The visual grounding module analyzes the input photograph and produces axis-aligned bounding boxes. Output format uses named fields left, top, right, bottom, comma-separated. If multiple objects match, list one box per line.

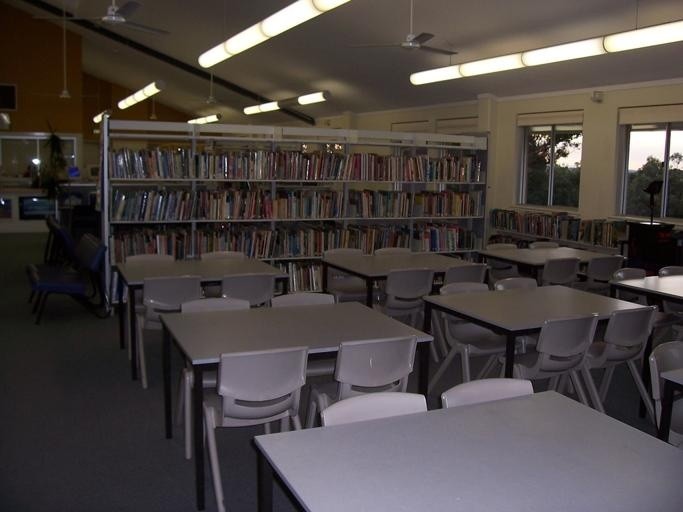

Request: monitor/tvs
left=0, top=84, right=17, bottom=112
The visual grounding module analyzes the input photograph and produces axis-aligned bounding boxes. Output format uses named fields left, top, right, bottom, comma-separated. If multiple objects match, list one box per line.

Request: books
left=109, top=141, right=489, bottom=291
left=489, top=207, right=627, bottom=249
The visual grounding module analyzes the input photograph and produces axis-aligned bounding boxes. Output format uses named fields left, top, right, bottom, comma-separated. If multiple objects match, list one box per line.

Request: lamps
left=116, top=78, right=167, bottom=111
left=59, top=13, right=73, bottom=99
left=243, top=99, right=280, bottom=116
left=297, top=89, right=330, bottom=106
left=185, top=113, right=223, bottom=124
left=196, top=1, right=351, bottom=70
left=408, top=0, right=682, bottom=88
left=93, top=108, right=113, bottom=124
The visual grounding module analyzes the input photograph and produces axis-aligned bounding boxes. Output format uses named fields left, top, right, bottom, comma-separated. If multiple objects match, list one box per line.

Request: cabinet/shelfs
left=487, top=207, right=628, bottom=276
left=104, top=113, right=489, bottom=305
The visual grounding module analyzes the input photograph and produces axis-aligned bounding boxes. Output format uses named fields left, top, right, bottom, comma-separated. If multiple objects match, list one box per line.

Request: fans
left=32, top=0, right=170, bottom=36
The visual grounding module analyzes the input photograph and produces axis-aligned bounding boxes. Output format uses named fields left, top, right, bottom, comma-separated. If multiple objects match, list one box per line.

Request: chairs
left=124, top=242, right=683, bottom=512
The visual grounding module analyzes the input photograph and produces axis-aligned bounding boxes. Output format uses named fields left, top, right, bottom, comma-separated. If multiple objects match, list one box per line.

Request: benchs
left=26, top=212, right=105, bottom=325
